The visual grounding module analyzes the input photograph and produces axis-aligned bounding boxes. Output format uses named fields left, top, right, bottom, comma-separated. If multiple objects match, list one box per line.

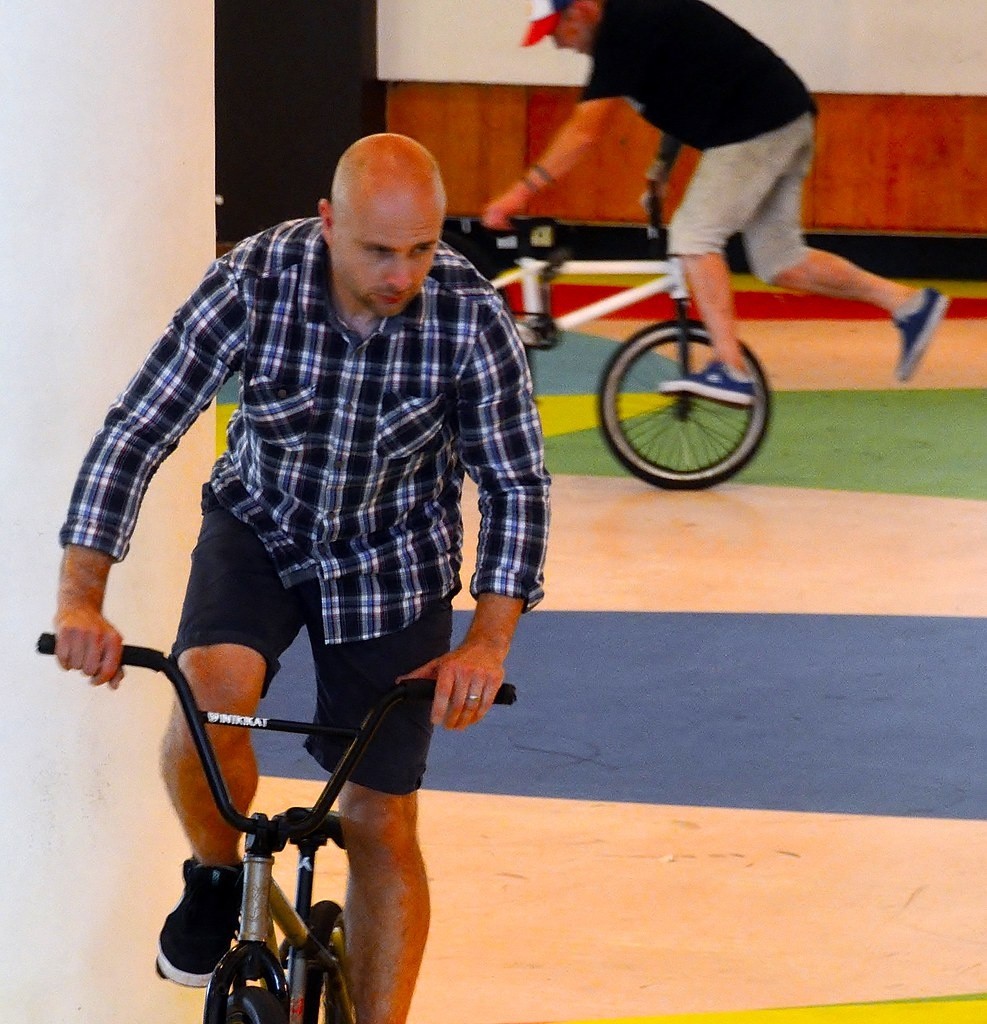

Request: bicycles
left=38, top=632, right=517, bottom=1024
left=482, top=181, right=771, bottom=490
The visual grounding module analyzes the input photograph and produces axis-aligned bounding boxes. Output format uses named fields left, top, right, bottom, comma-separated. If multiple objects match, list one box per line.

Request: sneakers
left=159, top=855, right=245, bottom=987
left=894, top=284, right=949, bottom=382
left=660, top=361, right=756, bottom=409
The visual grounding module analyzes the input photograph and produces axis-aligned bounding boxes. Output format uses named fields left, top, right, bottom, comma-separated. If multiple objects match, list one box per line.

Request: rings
left=467, top=695, right=482, bottom=702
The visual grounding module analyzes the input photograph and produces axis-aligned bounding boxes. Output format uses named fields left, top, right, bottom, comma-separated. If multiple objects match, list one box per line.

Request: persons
left=481, top=0, right=948, bottom=409
left=52, top=132, right=553, bottom=1024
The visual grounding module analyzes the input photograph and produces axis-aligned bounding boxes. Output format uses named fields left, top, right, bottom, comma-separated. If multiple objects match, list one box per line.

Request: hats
left=520, top=0, right=576, bottom=48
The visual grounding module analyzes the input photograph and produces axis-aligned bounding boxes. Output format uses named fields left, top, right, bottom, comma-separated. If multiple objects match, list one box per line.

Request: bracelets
left=519, top=174, right=540, bottom=194
left=531, top=163, right=557, bottom=184
